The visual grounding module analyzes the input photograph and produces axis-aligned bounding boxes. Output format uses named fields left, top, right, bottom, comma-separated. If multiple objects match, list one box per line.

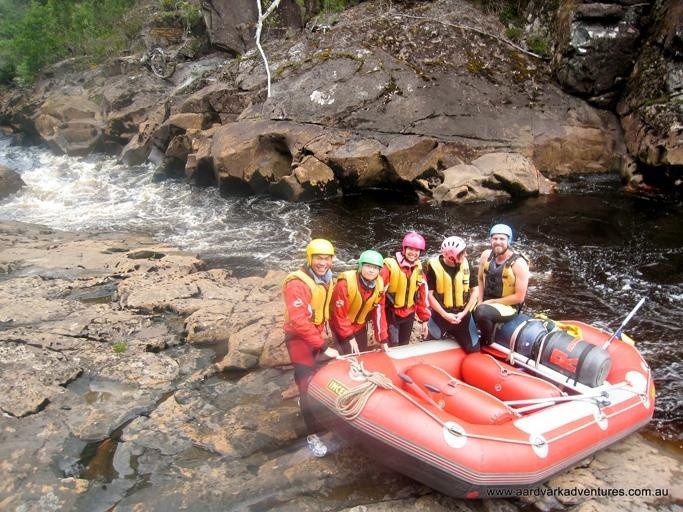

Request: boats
left=308, top=312, right=657, bottom=502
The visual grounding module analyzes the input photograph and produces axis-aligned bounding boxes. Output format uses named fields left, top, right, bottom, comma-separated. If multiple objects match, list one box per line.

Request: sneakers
left=307, top=434, right=327, bottom=457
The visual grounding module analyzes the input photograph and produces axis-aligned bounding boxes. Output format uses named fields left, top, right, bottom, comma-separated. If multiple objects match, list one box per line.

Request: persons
left=283, top=237, right=343, bottom=458
left=422, top=234, right=479, bottom=357
left=471, top=222, right=530, bottom=348
left=380, top=230, right=433, bottom=348
left=328, top=250, right=391, bottom=356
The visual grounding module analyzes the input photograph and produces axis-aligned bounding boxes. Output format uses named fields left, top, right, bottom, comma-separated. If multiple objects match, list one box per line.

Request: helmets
left=358, top=250, right=384, bottom=273
left=490, top=224, right=512, bottom=247
left=441, top=236, right=466, bottom=264
left=402, top=232, right=426, bottom=257
left=307, top=239, right=334, bottom=266
